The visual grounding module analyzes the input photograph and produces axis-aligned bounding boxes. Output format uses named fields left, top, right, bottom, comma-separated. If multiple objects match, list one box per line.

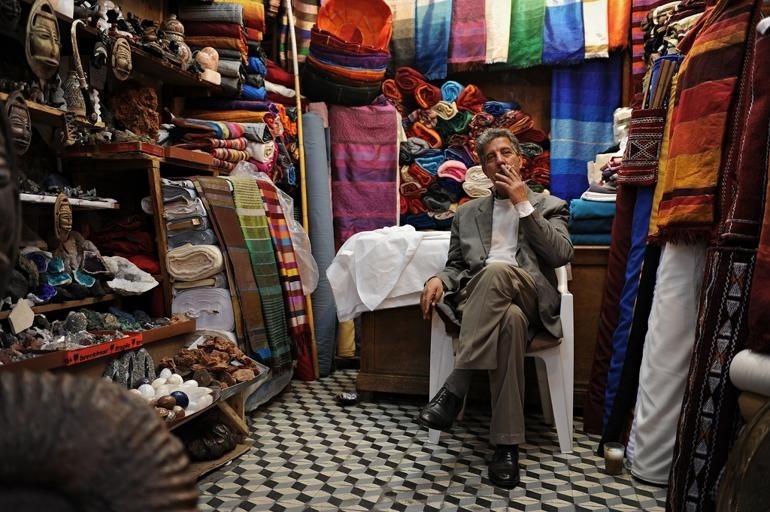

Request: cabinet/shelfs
left=0, top=0, right=309, bottom=319
left=352, top=235, right=611, bottom=416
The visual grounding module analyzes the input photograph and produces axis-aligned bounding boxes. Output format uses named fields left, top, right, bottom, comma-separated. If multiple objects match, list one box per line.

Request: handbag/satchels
left=615, top=55, right=685, bottom=185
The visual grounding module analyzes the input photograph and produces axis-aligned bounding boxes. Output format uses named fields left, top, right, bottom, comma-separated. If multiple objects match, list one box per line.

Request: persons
left=416, top=129, right=578, bottom=488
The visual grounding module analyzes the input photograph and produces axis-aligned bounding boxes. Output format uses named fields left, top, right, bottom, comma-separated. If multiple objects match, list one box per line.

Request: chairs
left=427, top=263, right=576, bottom=456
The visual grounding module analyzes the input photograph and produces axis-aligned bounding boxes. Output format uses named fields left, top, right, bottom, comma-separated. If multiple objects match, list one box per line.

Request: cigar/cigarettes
left=507, top=165, right=514, bottom=170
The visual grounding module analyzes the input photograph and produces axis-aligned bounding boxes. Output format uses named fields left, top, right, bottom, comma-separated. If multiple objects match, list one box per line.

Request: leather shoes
left=418, top=386, right=464, bottom=432
left=489, top=448, right=519, bottom=489
